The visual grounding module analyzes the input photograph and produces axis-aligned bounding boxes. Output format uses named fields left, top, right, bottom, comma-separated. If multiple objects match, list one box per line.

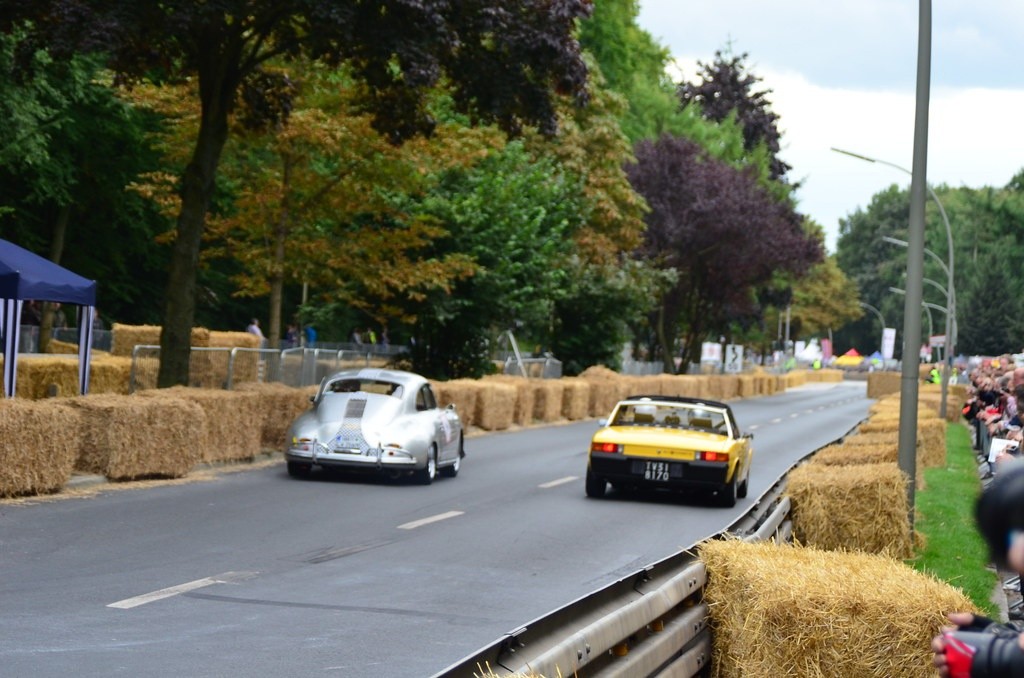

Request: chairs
left=633, top=413, right=653, bottom=422
left=690, top=417, right=713, bottom=428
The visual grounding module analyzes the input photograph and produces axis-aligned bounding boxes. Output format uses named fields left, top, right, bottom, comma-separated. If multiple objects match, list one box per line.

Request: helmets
left=687, top=402, right=711, bottom=426
left=633, top=396, right=654, bottom=422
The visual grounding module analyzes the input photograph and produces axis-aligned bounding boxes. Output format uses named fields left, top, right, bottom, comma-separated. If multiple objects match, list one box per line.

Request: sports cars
left=283, top=367, right=463, bottom=485
left=584, top=394, right=754, bottom=508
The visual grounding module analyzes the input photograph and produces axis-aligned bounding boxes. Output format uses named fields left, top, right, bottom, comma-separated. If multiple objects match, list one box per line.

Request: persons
left=20, top=293, right=112, bottom=360
left=923, top=346, right=1024, bottom=678
left=242, top=316, right=385, bottom=366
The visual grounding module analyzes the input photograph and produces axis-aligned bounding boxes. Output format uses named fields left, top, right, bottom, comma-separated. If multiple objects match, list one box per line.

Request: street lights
left=830, top=147, right=955, bottom=418
left=849, top=234, right=958, bottom=397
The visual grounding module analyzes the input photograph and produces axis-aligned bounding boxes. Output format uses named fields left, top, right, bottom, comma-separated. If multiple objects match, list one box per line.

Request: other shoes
left=969, top=427, right=1023, bottom=622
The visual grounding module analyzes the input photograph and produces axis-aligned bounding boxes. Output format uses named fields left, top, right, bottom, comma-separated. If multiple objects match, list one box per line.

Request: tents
left=0, top=236, right=97, bottom=399
